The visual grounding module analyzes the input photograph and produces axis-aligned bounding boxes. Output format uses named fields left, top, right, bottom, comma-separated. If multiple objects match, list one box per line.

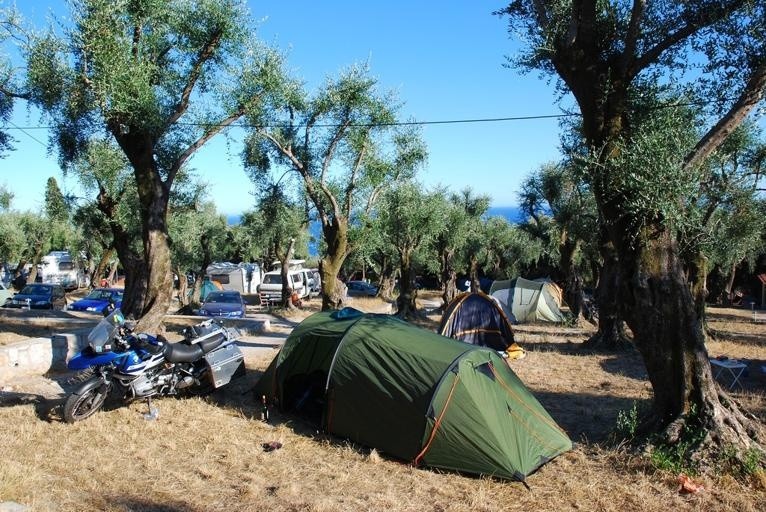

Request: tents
left=251, top=307, right=576, bottom=481
left=187, top=280, right=225, bottom=302
left=437, top=292, right=523, bottom=358
left=483, top=276, right=572, bottom=323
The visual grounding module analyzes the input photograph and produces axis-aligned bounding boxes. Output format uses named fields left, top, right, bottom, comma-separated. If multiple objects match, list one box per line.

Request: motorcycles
left=63, top=298, right=246, bottom=422
left=12, top=268, right=29, bottom=287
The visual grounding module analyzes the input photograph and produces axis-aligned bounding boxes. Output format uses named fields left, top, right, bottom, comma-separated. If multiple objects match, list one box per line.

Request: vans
left=259, top=260, right=315, bottom=303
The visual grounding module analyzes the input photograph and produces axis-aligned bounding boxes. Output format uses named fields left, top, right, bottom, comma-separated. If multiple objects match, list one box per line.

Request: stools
left=710, top=357, right=746, bottom=391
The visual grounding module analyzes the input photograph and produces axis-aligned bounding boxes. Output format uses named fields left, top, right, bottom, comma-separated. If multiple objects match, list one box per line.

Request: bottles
left=261, top=395, right=269, bottom=423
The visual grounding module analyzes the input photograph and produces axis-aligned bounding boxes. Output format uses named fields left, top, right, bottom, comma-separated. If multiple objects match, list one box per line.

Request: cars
left=199, top=290, right=248, bottom=318
left=346, top=281, right=377, bottom=297
left=0, top=281, right=124, bottom=312
